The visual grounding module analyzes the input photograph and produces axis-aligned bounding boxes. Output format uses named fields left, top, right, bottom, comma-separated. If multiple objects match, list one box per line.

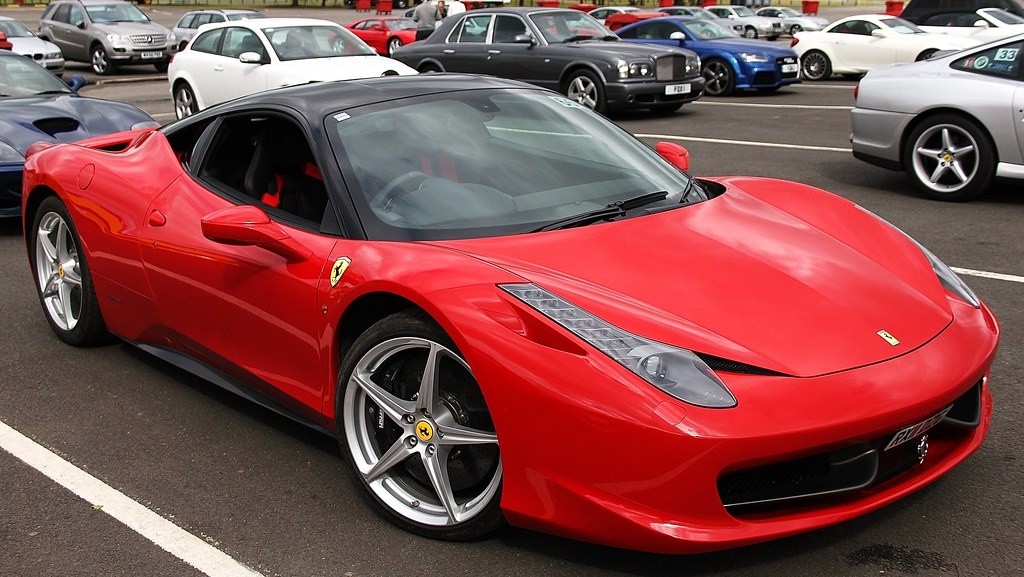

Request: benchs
left=211, top=34, right=278, bottom=57
left=455, top=22, right=490, bottom=42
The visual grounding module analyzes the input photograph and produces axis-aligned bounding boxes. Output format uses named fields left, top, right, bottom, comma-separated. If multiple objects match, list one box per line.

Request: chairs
left=245, top=114, right=329, bottom=223
left=645, top=25, right=662, bottom=38
left=536, top=18, right=558, bottom=43
left=851, top=22, right=869, bottom=35
left=281, top=30, right=315, bottom=57
left=389, top=100, right=484, bottom=182
left=499, top=22, right=523, bottom=43
left=236, top=33, right=263, bottom=58
left=838, top=24, right=851, bottom=33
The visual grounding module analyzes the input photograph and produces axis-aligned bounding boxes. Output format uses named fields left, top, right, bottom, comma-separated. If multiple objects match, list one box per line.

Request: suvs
left=163, top=18, right=424, bottom=137
left=38, top=0, right=179, bottom=76
left=170, top=10, right=269, bottom=54
left=1011, top=20, right=1012, bottom=21
left=703, top=6, right=775, bottom=40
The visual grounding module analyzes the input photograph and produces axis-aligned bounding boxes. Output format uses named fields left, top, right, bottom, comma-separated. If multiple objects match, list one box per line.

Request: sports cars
left=790, top=14, right=982, bottom=84
left=0, top=49, right=164, bottom=223
left=14, top=81, right=1000, bottom=555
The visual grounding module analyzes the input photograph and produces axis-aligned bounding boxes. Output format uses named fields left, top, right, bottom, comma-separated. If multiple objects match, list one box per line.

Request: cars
left=850, top=27, right=1024, bottom=194
left=659, top=5, right=741, bottom=39
left=593, top=23, right=804, bottom=96
left=329, top=16, right=434, bottom=58
left=407, top=18, right=709, bottom=119
left=756, top=7, right=828, bottom=39
left=397, top=5, right=424, bottom=20
left=0, top=15, right=66, bottom=80
left=549, top=5, right=663, bottom=38
left=899, top=0, right=1024, bottom=32
